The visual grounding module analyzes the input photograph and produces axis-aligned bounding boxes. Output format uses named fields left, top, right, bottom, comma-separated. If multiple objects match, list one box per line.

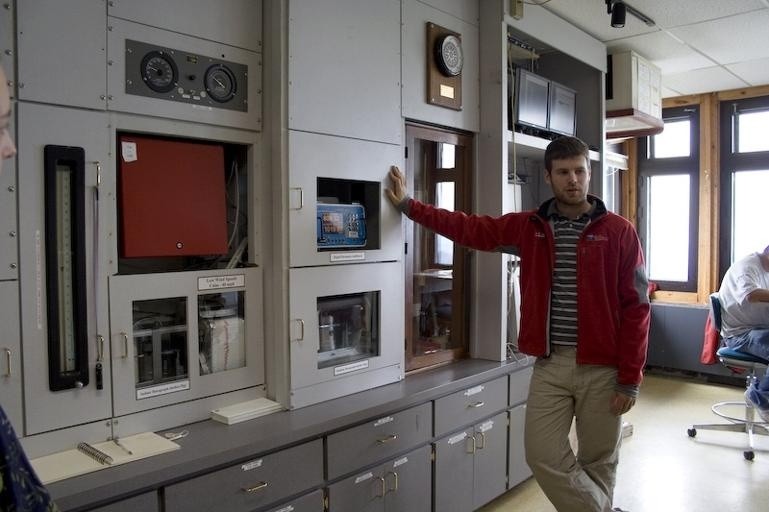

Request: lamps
left=605, top=0, right=625, bottom=29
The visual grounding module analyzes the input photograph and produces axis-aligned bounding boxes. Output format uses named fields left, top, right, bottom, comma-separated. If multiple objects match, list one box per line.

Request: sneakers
left=743, top=388, right=769, bottom=424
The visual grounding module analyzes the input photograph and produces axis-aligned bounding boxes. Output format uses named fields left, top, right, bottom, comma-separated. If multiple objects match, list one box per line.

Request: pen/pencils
left=114, top=439, right=133, bottom=456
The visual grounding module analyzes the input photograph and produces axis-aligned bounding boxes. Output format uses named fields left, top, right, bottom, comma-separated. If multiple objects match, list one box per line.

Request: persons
left=384, top=133, right=653, bottom=511
left=717, top=242, right=769, bottom=427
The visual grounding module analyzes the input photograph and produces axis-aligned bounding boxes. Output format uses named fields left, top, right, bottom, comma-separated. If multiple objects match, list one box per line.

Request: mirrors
left=405, top=121, right=473, bottom=376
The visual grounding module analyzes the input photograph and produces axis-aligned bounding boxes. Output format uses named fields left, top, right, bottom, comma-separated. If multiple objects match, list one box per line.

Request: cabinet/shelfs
left=15, top=100, right=270, bottom=462
left=30, top=360, right=535, bottom=511
left=472, top=21, right=606, bottom=360
left=265, top=127, right=406, bottom=415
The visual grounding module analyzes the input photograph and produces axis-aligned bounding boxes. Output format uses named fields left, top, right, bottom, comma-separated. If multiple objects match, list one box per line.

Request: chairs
left=688, top=293, right=768, bottom=461
